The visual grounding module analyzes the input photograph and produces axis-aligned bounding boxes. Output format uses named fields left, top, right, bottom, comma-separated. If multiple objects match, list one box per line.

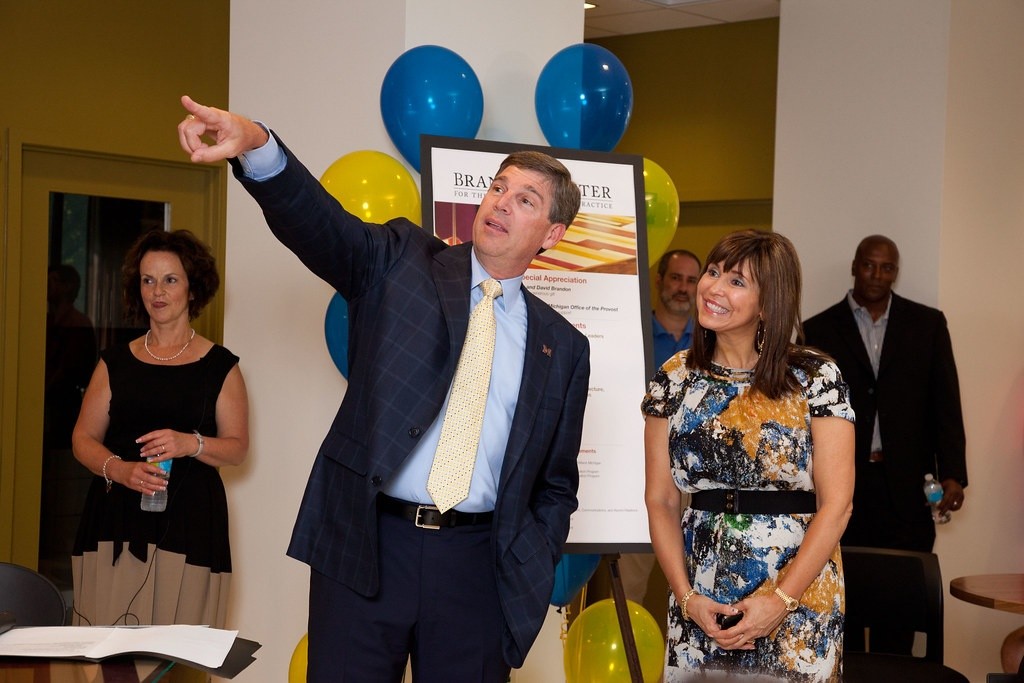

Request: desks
left=950, top=572, right=1024, bottom=683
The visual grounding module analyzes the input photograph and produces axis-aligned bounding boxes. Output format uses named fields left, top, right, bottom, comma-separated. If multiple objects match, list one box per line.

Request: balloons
left=550, top=552, right=600, bottom=614
left=534, top=44, right=634, bottom=152
left=643, top=153, right=679, bottom=271
left=320, top=149, right=422, bottom=230
left=288, top=634, right=309, bottom=683
left=325, top=292, right=350, bottom=382
left=380, top=45, right=484, bottom=175
left=563, top=599, right=664, bottom=683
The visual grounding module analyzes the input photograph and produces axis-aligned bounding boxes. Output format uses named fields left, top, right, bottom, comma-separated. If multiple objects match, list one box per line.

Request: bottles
left=924, top=474, right=952, bottom=525
left=140, top=453, right=172, bottom=512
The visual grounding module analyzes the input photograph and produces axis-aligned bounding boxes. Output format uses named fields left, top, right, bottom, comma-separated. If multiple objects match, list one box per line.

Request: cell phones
left=721, top=611, right=744, bottom=631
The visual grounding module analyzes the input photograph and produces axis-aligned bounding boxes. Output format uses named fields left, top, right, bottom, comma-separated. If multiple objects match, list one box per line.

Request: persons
left=178, top=93, right=591, bottom=683
left=652, top=249, right=702, bottom=373
left=48, top=262, right=97, bottom=378
left=72, top=234, right=249, bottom=626
left=641, top=230, right=858, bottom=683
left=796, top=233, right=968, bottom=656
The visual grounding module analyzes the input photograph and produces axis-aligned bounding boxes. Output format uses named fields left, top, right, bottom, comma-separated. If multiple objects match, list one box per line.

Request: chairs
left=0, top=561, right=66, bottom=629
left=839, top=546, right=971, bottom=683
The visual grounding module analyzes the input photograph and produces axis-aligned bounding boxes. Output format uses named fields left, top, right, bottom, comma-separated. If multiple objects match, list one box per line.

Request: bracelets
left=190, top=430, right=203, bottom=457
left=681, top=589, right=699, bottom=621
left=103, top=455, right=121, bottom=493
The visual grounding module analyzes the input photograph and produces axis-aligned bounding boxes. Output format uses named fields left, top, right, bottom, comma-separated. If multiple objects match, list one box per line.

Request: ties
left=426, top=277, right=503, bottom=515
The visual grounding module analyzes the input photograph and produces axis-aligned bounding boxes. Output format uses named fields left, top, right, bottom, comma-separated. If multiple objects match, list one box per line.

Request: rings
left=954, top=502, right=957, bottom=504
left=162, top=445, right=164, bottom=450
left=140, top=480, right=144, bottom=484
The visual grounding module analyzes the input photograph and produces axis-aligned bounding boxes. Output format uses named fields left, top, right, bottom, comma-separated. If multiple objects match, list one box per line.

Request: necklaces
left=145, top=329, right=195, bottom=361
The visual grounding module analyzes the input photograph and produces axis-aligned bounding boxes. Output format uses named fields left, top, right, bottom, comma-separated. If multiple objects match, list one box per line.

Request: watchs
left=775, top=587, right=799, bottom=612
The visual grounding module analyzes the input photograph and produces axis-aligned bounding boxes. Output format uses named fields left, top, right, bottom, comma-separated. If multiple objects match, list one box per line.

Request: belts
left=870, top=451, right=882, bottom=465
left=376, top=494, right=496, bottom=529
left=689, top=487, right=820, bottom=516
